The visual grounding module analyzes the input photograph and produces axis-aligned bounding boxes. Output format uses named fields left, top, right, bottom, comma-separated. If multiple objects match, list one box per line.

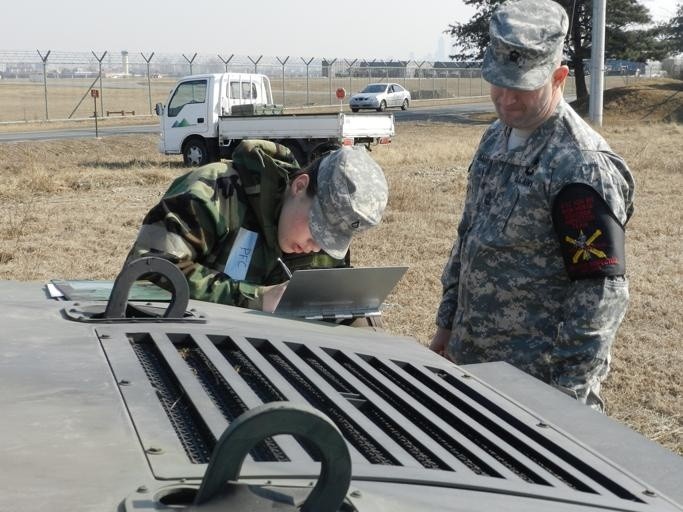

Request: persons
left=125, top=136, right=391, bottom=320
left=429, top=0, right=639, bottom=418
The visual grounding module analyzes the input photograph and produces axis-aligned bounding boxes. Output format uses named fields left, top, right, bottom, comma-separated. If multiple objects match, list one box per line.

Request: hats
left=481, top=0, right=571, bottom=92
left=308, top=147, right=390, bottom=259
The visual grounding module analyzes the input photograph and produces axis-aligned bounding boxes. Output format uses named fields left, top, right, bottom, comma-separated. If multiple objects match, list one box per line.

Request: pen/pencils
left=277, top=256, right=293, bottom=279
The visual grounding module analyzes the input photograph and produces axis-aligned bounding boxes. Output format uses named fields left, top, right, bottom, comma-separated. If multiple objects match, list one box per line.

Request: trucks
left=154, top=72, right=395, bottom=167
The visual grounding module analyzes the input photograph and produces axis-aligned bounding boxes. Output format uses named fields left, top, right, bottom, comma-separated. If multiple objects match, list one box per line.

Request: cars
left=349, top=82, right=412, bottom=113
